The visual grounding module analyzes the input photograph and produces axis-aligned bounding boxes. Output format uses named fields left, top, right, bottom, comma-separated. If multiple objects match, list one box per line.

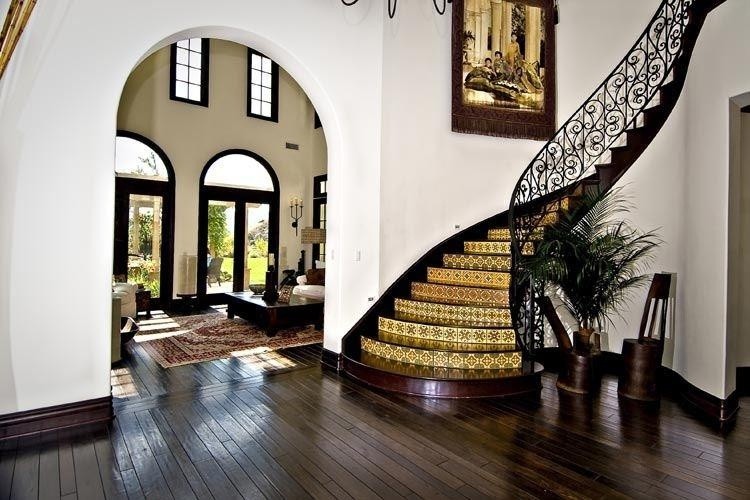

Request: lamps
left=289, top=197, right=305, bottom=235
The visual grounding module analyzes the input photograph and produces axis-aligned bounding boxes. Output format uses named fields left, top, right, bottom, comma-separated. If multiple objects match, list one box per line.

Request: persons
left=481, top=32, right=534, bottom=90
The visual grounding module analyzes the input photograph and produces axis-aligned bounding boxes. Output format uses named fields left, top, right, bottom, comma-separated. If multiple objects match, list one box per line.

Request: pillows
left=305, top=267, right=325, bottom=286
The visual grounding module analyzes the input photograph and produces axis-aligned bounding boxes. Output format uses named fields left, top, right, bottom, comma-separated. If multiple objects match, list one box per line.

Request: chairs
left=292, top=260, right=326, bottom=314
left=113, top=274, right=139, bottom=322
left=206, top=257, right=224, bottom=287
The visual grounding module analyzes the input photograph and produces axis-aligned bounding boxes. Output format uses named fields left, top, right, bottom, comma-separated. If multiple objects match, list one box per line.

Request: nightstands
left=135, top=289, right=152, bottom=319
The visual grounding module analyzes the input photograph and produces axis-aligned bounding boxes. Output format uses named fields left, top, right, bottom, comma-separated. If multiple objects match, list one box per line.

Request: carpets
left=132, top=309, right=324, bottom=369
